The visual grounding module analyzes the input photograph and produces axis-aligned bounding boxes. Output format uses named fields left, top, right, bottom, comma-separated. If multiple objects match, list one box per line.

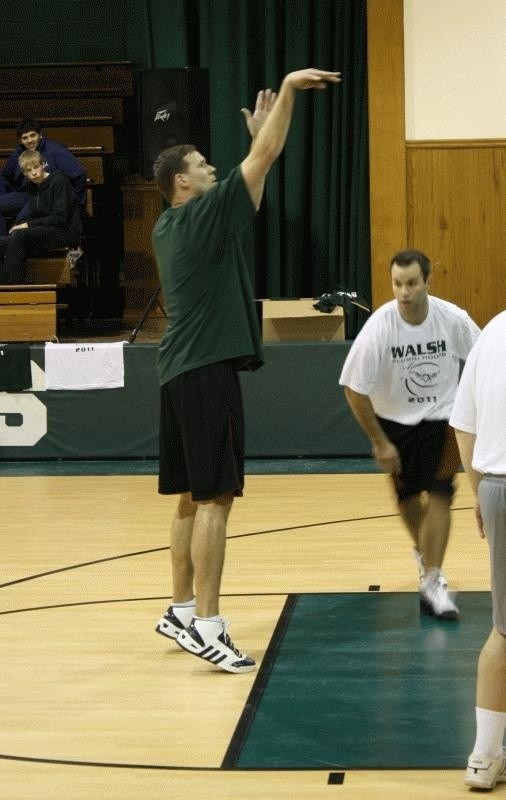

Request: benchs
left=0, top=62, right=138, bottom=333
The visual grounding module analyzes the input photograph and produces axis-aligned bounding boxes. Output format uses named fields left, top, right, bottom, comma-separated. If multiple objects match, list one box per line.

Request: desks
left=0, top=341, right=375, bottom=459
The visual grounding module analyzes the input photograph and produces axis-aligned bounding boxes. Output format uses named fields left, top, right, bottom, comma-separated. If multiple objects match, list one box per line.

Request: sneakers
left=419, top=552, right=459, bottom=620
left=465, top=754, right=506, bottom=789
left=156, top=599, right=256, bottom=673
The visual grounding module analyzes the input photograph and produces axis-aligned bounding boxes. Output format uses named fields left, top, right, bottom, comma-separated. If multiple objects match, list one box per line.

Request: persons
left=445, top=304, right=505, bottom=799
left=334, top=246, right=480, bottom=624
left=148, top=68, right=345, bottom=675
left=0, top=150, right=82, bottom=285
left=0, top=116, right=86, bottom=245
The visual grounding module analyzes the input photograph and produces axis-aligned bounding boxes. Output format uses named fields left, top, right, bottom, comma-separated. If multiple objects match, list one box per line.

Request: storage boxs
left=252, top=293, right=347, bottom=342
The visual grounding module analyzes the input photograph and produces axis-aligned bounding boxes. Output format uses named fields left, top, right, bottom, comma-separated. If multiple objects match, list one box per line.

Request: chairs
left=0, top=291, right=57, bottom=341
